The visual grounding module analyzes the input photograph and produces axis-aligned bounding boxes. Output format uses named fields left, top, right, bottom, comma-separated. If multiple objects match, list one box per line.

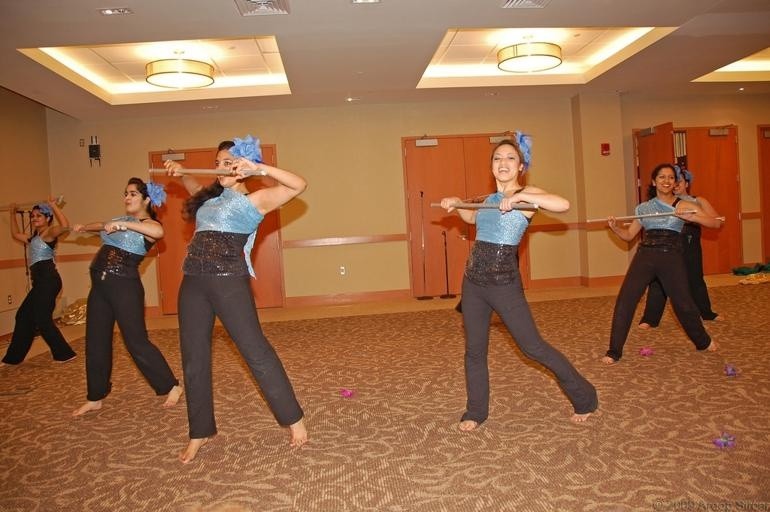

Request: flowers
left=37, top=202, right=53, bottom=215
left=147, top=181, right=170, bottom=209
left=515, top=130, right=533, bottom=175
left=228, top=134, right=263, bottom=166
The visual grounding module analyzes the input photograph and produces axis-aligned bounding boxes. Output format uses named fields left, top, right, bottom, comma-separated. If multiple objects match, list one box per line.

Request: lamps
left=496, top=41, right=564, bottom=74
left=145, top=60, right=215, bottom=91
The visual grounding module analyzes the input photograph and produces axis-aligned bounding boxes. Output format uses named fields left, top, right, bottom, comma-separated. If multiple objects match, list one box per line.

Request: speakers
left=88, top=144, right=101, bottom=161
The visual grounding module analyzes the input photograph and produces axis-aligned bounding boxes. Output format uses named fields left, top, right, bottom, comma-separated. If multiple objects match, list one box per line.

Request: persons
left=73, top=176, right=182, bottom=415
left=601, top=163, right=721, bottom=364
left=0, top=196, right=77, bottom=364
left=441, top=139, right=598, bottom=433
left=161, top=141, right=308, bottom=463
